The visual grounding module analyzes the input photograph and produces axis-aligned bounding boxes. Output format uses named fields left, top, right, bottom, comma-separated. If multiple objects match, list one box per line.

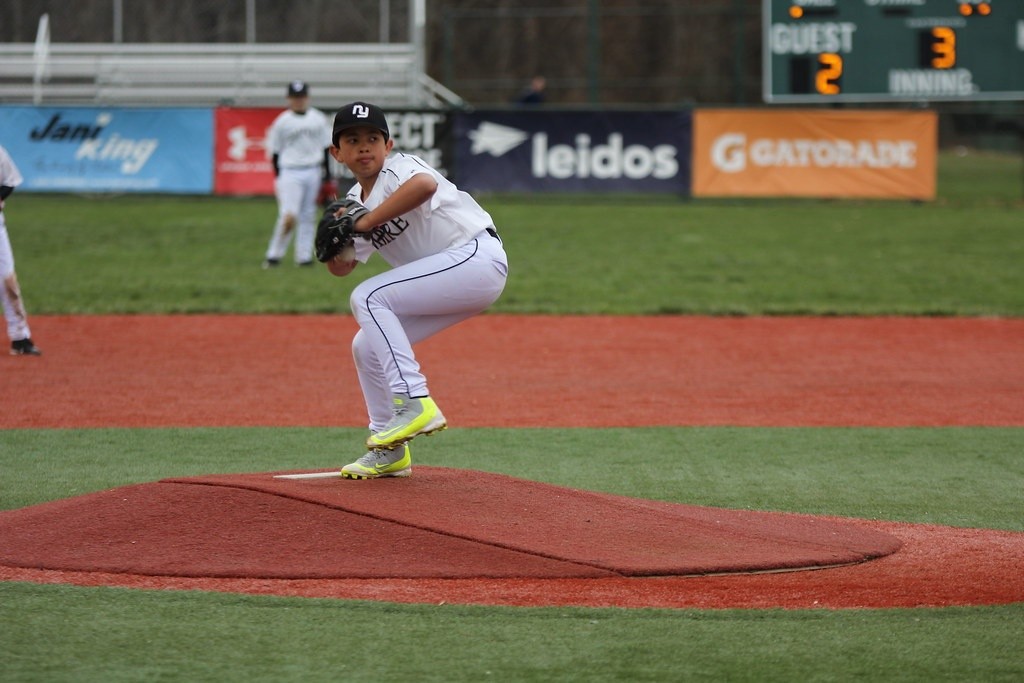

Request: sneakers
left=339, top=442, right=414, bottom=480
left=368, top=392, right=447, bottom=452
left=10, top=339, right=40, bottom=356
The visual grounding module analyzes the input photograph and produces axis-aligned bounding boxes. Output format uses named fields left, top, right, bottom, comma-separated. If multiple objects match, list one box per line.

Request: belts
left=488, top=226, right=501, bottom=241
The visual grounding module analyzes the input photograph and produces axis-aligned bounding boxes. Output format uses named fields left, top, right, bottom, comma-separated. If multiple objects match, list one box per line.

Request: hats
left=285, top=80, right=308, bottom=99
left=332, top=102, right=389, bottom=148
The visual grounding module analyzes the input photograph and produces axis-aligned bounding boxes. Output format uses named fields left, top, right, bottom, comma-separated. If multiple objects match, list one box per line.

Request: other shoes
left=299, top=260, right=313, bottom=266
left=262, top=257, right=281, bottom=267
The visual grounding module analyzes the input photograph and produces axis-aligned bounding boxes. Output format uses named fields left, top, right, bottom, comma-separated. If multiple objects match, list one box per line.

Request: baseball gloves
left=314, top=198, right=380, bottom=264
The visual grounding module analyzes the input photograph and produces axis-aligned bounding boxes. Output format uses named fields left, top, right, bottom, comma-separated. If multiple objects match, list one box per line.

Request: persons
left=314, top=101, right=509, bottom=480
left=0, top=143, right=41, bottom=355
left=262, top=82, right=332, bottom=265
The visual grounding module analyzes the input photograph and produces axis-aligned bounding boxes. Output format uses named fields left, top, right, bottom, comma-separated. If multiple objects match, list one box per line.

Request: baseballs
left=340, top=245, right=357, bottom=263
